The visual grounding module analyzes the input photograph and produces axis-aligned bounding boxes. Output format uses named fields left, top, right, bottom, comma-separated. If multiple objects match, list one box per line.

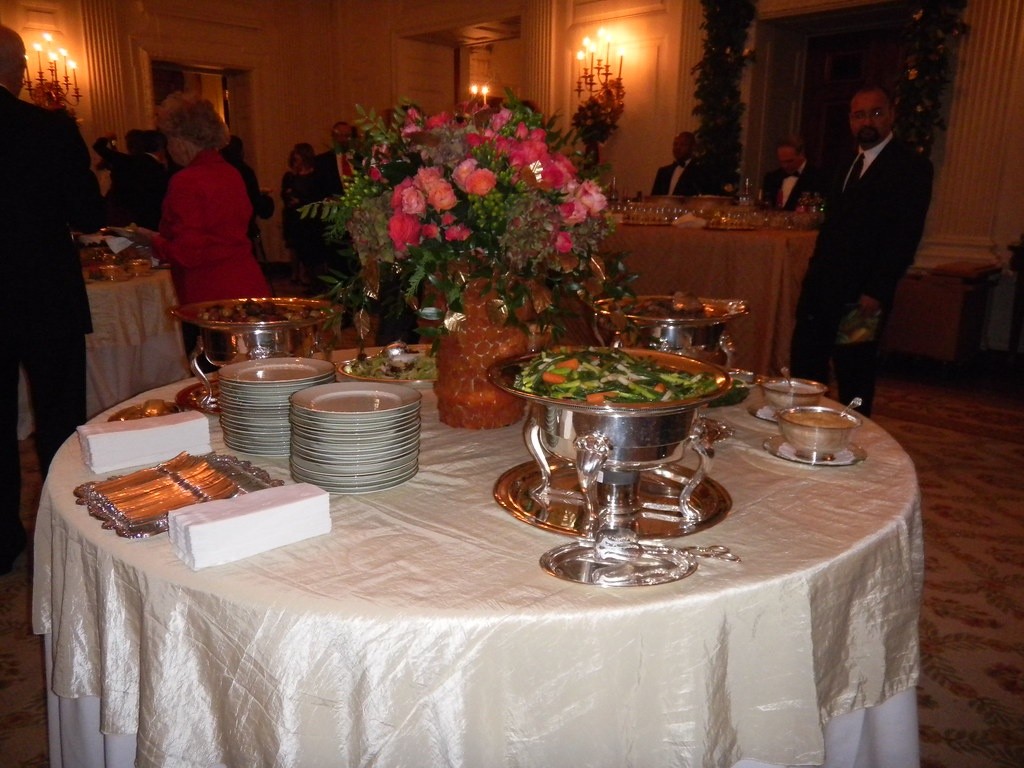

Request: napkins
left=77, top=406, right=212, bottom=475
left=167, top=481, right=331, bottom=573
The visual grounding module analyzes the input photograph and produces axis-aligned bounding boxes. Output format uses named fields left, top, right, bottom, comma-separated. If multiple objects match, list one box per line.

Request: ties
left=843, top=153, right=865, bottom=195
left=342, top=154, right=353, bottom=179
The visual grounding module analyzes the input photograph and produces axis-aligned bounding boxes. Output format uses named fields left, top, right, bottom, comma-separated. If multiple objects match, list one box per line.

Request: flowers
left=295, top=85, right=647, bottom=357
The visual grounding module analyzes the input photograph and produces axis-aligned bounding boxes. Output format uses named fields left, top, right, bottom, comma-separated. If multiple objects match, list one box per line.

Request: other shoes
left=0, top=522, right=27, bottom=576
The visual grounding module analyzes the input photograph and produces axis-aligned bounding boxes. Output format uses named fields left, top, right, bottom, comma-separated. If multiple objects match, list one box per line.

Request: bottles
left=738, top=177, right=754, bottom=207
left=606, top=176, right=620, bottom=203
left=796, top=193, right=823, bottom=212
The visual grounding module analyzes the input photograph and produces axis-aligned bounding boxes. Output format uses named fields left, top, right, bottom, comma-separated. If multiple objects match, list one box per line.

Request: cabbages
left=351, top=351, right=439, bottom=380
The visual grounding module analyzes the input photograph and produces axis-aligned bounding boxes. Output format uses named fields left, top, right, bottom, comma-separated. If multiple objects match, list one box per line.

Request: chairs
left=538, top=250, right=610, bottom=346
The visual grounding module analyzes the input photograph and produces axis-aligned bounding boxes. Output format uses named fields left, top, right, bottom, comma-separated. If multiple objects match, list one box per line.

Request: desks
left=880, top=259, right=1001, bottom=371
left=594, top=221, right=819, bottom=378
left=27, top=342, right=925, bottom=768
left=17, top=268, right=193, bottom=441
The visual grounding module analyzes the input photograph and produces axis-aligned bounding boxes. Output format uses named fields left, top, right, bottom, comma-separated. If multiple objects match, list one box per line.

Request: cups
left=600, top=199, right=817, bottom=231
left=86, top=247, right=152, bottom=282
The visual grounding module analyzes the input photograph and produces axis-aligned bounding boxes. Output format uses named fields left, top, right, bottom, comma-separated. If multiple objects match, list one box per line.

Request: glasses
left=849, top=109, right=889, bottom=121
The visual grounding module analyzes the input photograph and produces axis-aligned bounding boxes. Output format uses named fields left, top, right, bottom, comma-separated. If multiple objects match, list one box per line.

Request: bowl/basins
left=417, top=307, right=444, bottom=321
left=778, top=407, right=862, bottom=462
left=756, top=377, right=828, bottom=412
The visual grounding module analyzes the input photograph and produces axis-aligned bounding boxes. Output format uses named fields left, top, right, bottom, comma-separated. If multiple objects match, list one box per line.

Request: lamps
left=455, top=83, right=488, bottom=124
left=23, top=34, right=84, bottom=111
left=574, top=28, right=625, bottom=107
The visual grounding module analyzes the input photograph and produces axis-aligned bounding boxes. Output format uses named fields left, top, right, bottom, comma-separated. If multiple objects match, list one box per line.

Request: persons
left=755, top=129, right=823, bottom=209
left=91, top=125, right=275, bottom=283
left=118, top=88, right=274, bottom=377
left=787, top=81, right=933, bottom=420
left=0, top=22, right=107, bottom=575
left=278, top=118, right=422, bottom=349
left=650, top=132, right=707, bottom=197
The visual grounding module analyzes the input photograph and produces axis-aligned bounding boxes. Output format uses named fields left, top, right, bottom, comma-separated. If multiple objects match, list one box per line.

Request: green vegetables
left=514, top=346, right=717, bottom=402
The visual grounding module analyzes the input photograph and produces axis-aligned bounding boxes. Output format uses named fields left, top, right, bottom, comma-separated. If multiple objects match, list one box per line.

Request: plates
left=219, top=357, right=336, bottom=460
left=107, top=226, right=136, bottom=238
left=289, top=383, right=422, bottom=493
left=695, top=417, right=735, bottom=444
left=339, top=343, right=438, bottom=385
left=107, top=402, right=185, bottom=424
left=747, top=401, right=783, bottom=423
left=762, top=435, right=866, bottom=465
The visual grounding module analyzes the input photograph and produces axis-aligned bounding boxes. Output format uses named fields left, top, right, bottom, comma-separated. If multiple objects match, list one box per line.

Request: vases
left=407, top=254, right=556, bottom=431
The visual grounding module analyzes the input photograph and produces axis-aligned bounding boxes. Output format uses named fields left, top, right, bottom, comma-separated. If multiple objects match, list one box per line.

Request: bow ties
left=674, top=160, right=685, bottom=168
left=783, top=170, right=801, bottom=178
